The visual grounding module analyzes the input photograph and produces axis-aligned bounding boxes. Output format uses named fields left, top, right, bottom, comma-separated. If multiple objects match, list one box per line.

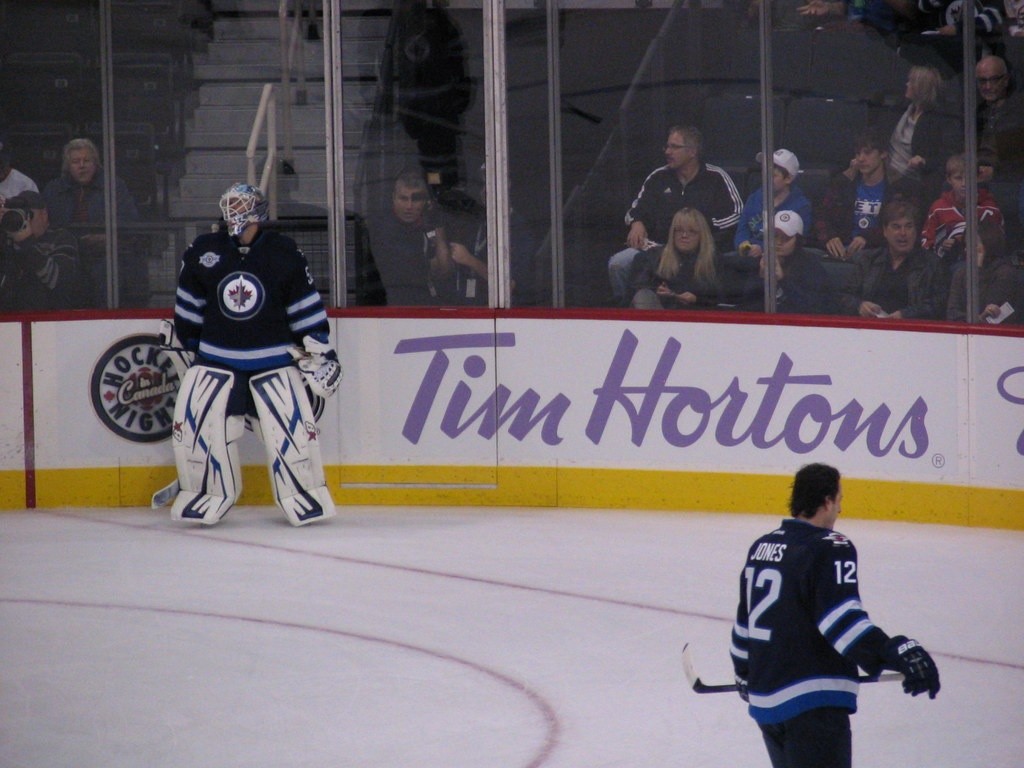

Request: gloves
left=888, top=635, right=940, bottom=699
left=736, top=676, right=749, bottom=704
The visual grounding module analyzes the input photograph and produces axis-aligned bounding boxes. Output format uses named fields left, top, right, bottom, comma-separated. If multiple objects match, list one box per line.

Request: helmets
left=219, top=182, right=269, bottom=237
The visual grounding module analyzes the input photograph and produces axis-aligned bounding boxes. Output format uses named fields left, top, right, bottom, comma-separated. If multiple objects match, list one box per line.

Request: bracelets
left=629, top=220, right=640, bottom=227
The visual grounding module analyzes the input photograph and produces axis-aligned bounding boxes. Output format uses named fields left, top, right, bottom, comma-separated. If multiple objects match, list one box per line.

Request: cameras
left=2, top=197, right=34, bottom=231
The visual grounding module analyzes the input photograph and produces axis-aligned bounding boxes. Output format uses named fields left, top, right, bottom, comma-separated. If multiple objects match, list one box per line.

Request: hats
left=757, top=148, right=799, bottom=178
left=774, top=209, right=803, bottom=236
left=18, top=190, right=54, bottom=209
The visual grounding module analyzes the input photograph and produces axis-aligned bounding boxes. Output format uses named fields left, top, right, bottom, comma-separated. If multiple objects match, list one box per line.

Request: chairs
left=0, top=0, right=211, bottom=313
left=367, top=0, right=1024, bottom=327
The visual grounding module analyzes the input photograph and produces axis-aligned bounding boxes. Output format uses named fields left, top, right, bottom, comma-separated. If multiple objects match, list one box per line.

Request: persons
left=732, top=148, right=811, bottom=272
left=374, top=168, right=458, bottom=309
left=884, top=61, right=946, bottom=207
left=361, top=1, right=471, bottom=211
left=1, top=136, right=89, bottom=310
left=166, top=182, right=345, bottom=528
left=41, top=137, right=155, bottom=307
left=449, top=161, right=534, bottom=307
left=725, top=0, right=1024, bottom=89
left=730, top=463, right=940, bottom=768
left=945, top=221, right=1024, bottom=325
left=958, top=55, right=1024, bottom=185
left=813, top=129, right=898, bottom=261
left=920, top=150, right=1005, bottom=257
left=745, top=208, right=841, bottom=313
left=841, top=197, right=948, bottom=322
left=620, top=206, right=721, bottom=310
left=606, top=121, right=744, bottom=307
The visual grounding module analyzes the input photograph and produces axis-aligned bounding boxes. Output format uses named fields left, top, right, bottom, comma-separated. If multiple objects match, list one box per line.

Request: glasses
left=978, top=73, right=1007, bottom=83
left=663, top=144, right=688, bottom=151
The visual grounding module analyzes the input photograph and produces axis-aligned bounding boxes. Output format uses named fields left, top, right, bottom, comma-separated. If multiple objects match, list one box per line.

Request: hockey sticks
left=680, top=644, right=905, bottom=694
left=151, top=477, right=180, bottom=508
